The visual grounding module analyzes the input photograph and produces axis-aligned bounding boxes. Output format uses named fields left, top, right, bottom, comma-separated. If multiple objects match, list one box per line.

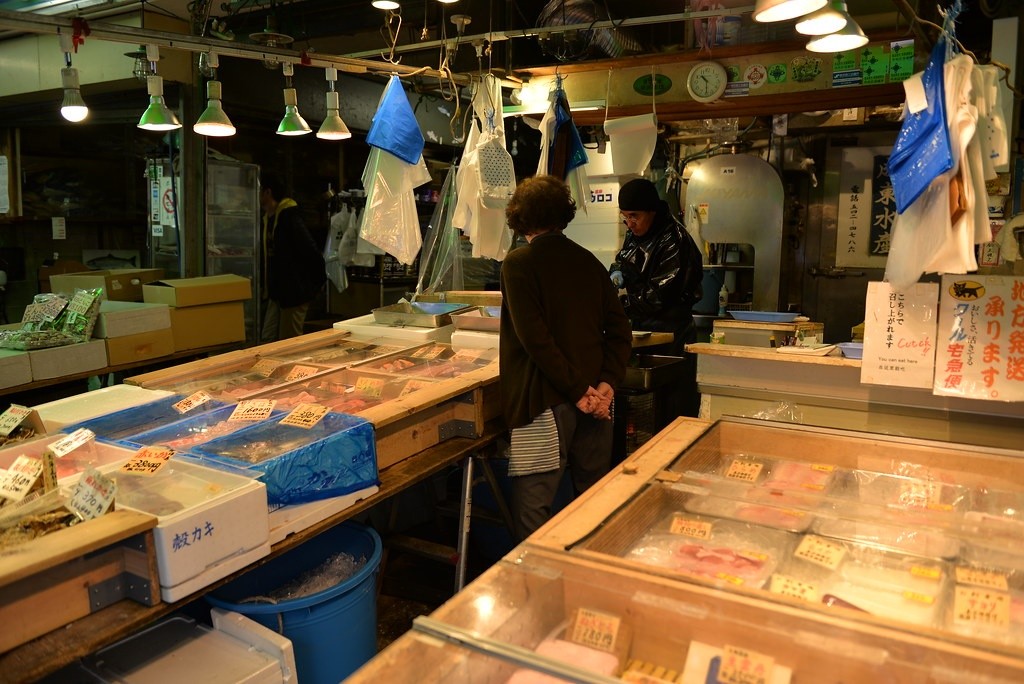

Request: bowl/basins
left=837, top=342, right=863, bottom=358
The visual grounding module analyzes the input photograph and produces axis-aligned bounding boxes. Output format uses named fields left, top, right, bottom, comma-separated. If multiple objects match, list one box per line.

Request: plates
left=728, top=310, right=801, bottom=322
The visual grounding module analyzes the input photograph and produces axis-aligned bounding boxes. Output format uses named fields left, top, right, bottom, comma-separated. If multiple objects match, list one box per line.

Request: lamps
left=751, top=1, right=869, bottom=53
left=316, top=67, right=352, bottom=141
left=249, top=14, right=294, bottom=48
left=193, top=49, right=236, bottom=137
left=275, top=62, right=312, bottom=136
left=137, top=44, right=183, bottom=131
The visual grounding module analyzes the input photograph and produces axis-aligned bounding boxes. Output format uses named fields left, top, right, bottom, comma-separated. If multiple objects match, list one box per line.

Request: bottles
left=718, top=284, right=728, bottom=316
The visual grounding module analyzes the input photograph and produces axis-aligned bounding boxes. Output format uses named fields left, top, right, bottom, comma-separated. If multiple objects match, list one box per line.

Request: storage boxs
left=0, top=268, right=498, bottom=656
left=79, top=613, right=284, bottom=684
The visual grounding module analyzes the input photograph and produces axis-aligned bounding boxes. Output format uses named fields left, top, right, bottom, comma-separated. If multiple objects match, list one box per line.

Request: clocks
left=686, top=61, right=728, bottom=103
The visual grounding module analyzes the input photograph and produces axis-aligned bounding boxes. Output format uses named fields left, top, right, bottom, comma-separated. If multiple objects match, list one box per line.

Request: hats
left=618, top=178, right=659, bottom=211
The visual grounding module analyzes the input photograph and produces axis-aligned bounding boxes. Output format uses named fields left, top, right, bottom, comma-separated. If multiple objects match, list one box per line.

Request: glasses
left=619, top=210, right=644, bottom=222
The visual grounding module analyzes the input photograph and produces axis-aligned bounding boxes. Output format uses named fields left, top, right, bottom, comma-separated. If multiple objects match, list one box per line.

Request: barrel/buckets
left=202, top=522, right=382, bottom=684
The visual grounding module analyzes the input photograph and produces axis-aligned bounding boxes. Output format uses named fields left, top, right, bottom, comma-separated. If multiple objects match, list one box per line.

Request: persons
left=498, top=175, right=634, bottom=542
left=607, top=179, right=704, bottom=433
left=260, top=178, right=327, bottom=343
